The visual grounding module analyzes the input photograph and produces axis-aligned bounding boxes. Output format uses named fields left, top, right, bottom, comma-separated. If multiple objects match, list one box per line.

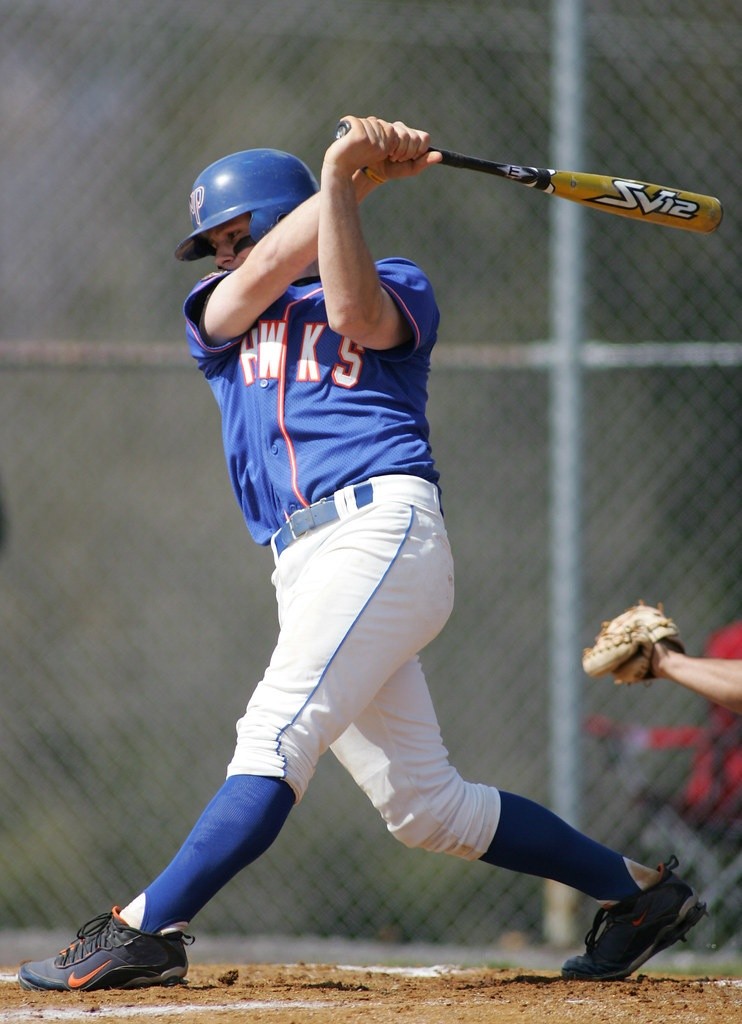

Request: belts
left=274, top=483, right=372, bottom=554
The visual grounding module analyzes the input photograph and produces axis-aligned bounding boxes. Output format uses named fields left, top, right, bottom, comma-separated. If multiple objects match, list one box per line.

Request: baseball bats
left=335, top=119, right=725, bottom=238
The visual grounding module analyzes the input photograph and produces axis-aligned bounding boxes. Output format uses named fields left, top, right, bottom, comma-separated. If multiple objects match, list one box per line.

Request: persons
left=616, top=640, right=742, bottom=713
left=22, top=113, right=713, bottom=988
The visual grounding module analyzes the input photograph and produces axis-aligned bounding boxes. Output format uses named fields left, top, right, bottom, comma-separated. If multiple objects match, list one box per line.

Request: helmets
left=174, top=146, right=318, bottom=263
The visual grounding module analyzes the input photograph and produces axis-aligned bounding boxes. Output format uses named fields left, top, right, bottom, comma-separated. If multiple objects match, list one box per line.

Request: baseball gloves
left=576, top=604, right=686, bottom=689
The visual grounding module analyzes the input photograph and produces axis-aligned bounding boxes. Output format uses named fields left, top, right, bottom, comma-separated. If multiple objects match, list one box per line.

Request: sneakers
left=560, top=854, right=710, bottom=982
left=18, top=906, right=194, bottom=991
